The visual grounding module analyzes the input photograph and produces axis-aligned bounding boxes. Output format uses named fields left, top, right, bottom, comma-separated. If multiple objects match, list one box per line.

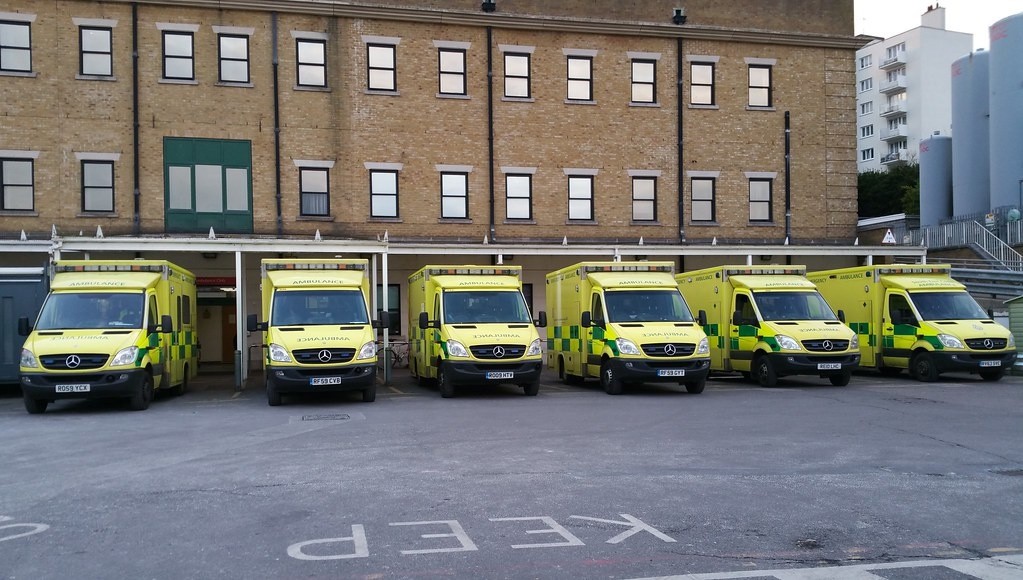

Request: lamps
left=202, top=253, right=217, bottom=259
left=761, top=255, right=772, bottom=261
left=635, top=255, right=647, bottom=261
left=934, top=131, right=940, bottom=135
left=359, top=253, right=373, bottom=260
left=503, top=255, right=513, bottom=261
left=672, top=15, right=688, bottom=25
left=481, top=2, right=496, bottom=13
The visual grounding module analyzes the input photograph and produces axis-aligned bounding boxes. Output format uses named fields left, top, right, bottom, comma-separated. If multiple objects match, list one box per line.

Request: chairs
left=326, top=297, right=358, bottom=323
left=447, top=294, right=470, bottom=322
left=277, top=295, right=309, bottom=323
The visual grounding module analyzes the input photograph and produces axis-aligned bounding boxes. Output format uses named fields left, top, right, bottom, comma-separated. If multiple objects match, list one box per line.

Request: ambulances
left=673, top=265, right=861, bottom=387
left=543, top=260, right=711, bottom=394
left=806, top=263, right=1018, bottom=382
left=407, top=265, right=547, bottom=398
left=18, top=258, right=197, bottom=415
left=247, top=258, right=391, bottom=407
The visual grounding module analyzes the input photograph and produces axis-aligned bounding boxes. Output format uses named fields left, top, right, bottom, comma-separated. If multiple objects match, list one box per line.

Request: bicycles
left=376, top=341, right=409, bottom=369
